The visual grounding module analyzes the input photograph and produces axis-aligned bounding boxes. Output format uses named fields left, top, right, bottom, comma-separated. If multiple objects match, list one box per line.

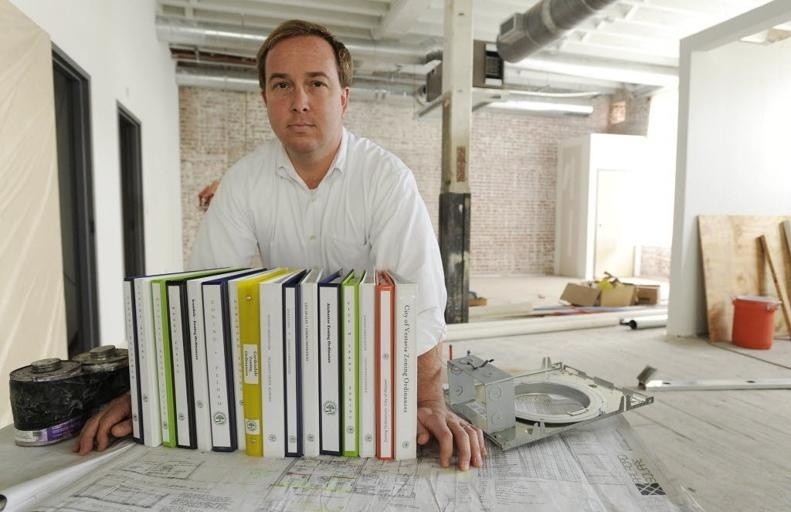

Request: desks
left=1, top=401, right=119, bottom=492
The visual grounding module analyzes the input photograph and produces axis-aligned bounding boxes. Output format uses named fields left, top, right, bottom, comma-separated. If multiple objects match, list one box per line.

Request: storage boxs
left=558, top=276, right=641, bottom=308
left=634, top=283, right=662, bottom=307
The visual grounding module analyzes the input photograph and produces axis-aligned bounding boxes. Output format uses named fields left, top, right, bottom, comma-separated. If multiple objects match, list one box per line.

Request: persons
left=66, top=17, right=488, bottom=471
